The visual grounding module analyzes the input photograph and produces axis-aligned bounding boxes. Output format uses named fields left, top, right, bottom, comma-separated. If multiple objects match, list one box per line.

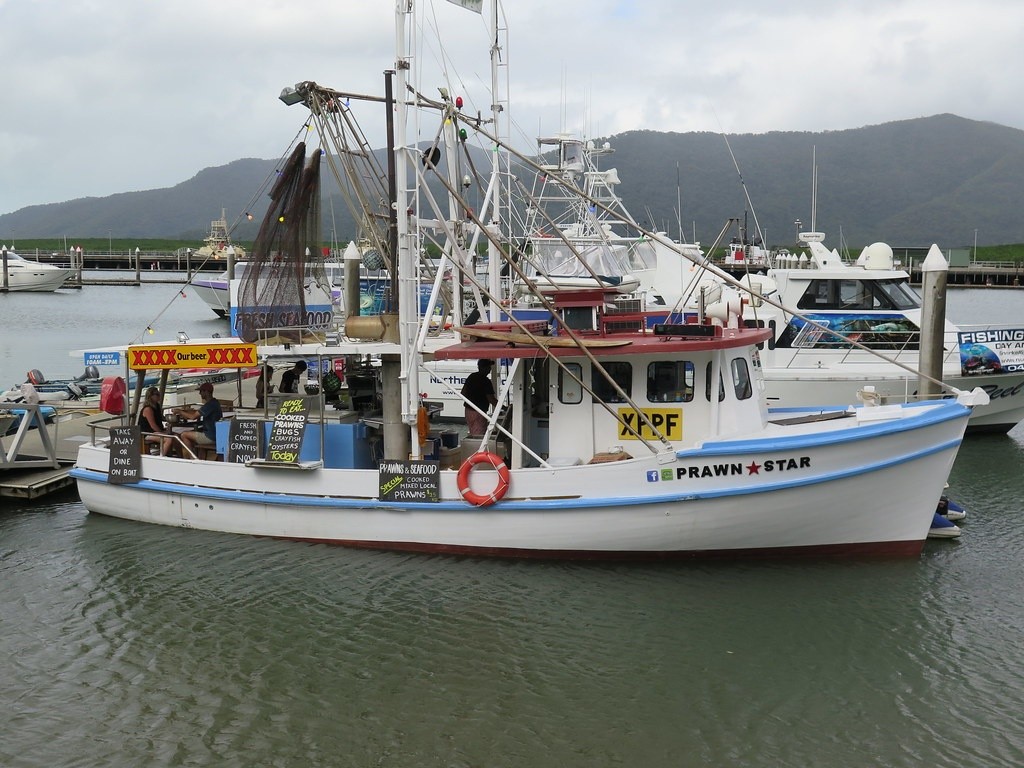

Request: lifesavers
left=455, top=451, right=509, bottom=507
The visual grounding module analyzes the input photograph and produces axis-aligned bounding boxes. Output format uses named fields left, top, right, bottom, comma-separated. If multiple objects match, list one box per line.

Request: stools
left=141, top=440, right=160, bottom=456
left=195, top=444, right=223, bottom=461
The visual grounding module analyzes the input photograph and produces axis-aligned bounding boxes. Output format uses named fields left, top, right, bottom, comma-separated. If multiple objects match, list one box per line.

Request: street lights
left=974, top=229, right=978, bottom=264
left=108, top=229, right=112, bottom=260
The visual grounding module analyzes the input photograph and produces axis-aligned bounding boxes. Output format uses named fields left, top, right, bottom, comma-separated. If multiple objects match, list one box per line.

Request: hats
left=195, top=382, right=213, bottom=393
left=477, top=358, right=496, bottom=367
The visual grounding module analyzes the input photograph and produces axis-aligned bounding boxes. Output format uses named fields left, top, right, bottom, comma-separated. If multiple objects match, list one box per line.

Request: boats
left=0, top=250, right=81, bottom=292
left=0, top=0, right=1024, bottom=558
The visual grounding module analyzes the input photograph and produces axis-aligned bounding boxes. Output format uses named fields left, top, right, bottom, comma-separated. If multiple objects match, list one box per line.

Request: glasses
left=151, top=393, right=160, bottom=396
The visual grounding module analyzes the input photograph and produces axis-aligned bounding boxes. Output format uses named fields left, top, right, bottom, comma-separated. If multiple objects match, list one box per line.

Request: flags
left=735, top=252, right=743, bottom=260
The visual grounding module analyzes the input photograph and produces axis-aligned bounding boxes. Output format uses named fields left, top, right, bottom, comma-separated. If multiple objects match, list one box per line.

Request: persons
left=278, top=360, right=307, bottom=393
left=461, top=358, right=507, bottom=436
left=255, top=366, right=275, bottom=408
left=136, top=387, right=173, bottom=457
left=173, top=382, right=222, bottom=459
